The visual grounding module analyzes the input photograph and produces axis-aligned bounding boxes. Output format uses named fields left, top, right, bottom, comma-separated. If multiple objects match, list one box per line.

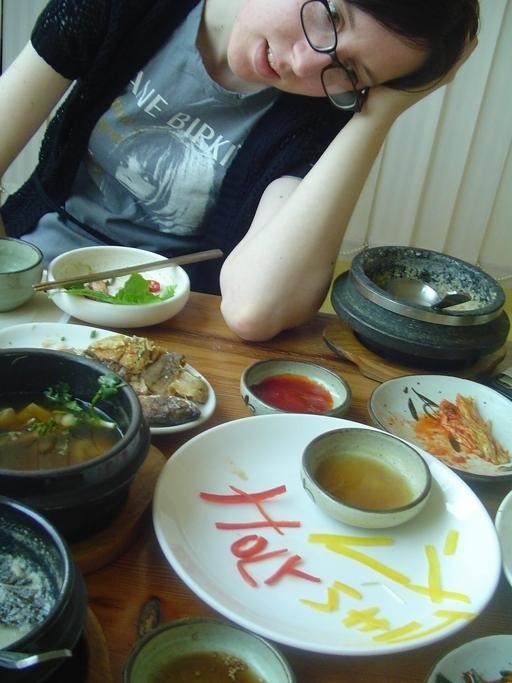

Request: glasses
left=300, top=0, right=367, bottom=113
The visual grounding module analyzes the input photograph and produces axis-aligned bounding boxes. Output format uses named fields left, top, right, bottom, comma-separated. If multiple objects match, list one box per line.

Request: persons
left=100, top=126, right=217, bottom=236
left=0, top=1, right=478, bottom=343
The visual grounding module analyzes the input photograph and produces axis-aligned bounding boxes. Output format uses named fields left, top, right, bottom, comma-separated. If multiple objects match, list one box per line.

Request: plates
left=427, top=635, right=512, bottom=683
left=151, top=413, right=502, bottom=658
left=0, top=322, right=216, bottom=435
left=367, top=375, right=512, bottom=482
left=495, top=490, right=512, bottom=588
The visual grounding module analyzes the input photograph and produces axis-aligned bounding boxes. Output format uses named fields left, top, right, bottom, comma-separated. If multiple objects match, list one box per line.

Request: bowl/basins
left=120, top=616, right=297, bottom=683
left=299, top=428, right=432, bottom=529
left=240, top=358, right=352, bottom=415
left=47, top=245, right=191, bottom=329
left=0, top=236, right=44, bottom=313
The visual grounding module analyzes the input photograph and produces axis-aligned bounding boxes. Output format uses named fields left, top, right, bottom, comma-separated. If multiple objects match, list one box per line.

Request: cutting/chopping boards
left=322, top=320, right=509, bottom=386
left=82, top=608, right=115, bottom=683
left=70, top=443, right=167, bottom=577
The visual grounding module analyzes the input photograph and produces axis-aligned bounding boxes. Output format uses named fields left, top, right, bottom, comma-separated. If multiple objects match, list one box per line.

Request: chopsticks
left=31, top=247, right=224, bottom=291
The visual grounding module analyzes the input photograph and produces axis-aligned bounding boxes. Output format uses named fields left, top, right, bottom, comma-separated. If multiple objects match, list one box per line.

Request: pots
left=0, top=495, right=87, bottom=683
left=331, top=245, right=510, bottom=370
left=0, top=347, right=150, bottom=545
